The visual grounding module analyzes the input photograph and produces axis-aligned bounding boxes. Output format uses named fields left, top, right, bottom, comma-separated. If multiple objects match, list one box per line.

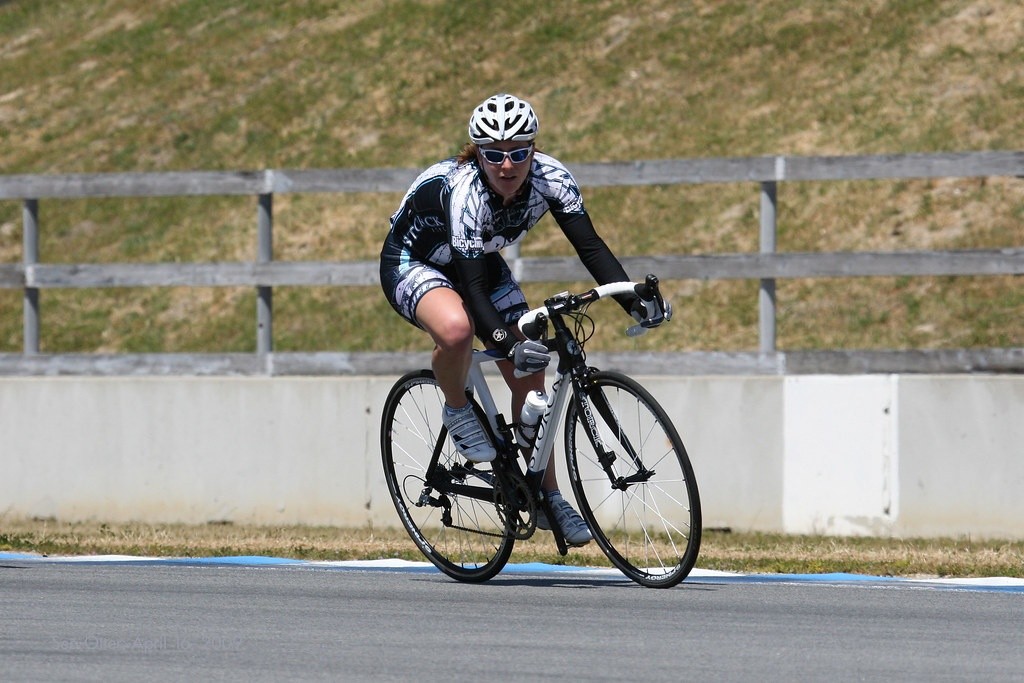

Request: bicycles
left=379, top=273, right=703, bottom=590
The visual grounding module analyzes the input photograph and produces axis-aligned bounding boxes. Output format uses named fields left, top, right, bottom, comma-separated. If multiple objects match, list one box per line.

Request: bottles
left=516, top=388, right=548, bottom=448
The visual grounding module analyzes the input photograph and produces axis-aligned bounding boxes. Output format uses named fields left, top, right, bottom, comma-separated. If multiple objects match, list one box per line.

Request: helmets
left=469, top=93, right=539, bottom=145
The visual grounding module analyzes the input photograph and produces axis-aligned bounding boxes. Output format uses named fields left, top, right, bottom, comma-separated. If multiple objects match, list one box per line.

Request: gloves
left=631, top=294, right=671, bottom=329
left=506, top=339, right=550, bottom=373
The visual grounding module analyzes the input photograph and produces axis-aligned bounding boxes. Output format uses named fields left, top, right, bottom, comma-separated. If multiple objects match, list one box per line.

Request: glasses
left=478, top=145, right=532, bottom=165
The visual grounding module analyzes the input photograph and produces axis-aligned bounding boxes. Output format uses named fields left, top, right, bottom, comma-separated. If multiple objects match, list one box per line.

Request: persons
left=380, top=93, right=673, bottom=543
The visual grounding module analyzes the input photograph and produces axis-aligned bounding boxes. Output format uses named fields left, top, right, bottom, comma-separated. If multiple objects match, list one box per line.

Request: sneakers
left=442, top=405, right=496, bottom=462
left=536, top=500, right=593, bottom=543
